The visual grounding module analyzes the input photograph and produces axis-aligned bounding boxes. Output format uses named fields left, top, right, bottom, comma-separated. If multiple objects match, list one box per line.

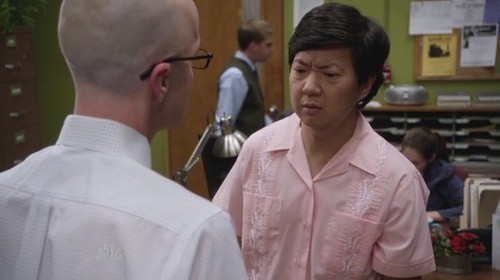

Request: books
left=459, top=177, right=500, bottom=229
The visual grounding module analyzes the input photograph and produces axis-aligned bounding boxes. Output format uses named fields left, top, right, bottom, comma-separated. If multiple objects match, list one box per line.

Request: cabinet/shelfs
left=360, top=104, right=500, bottom=162
left=0, top=25, right=41, bottom=174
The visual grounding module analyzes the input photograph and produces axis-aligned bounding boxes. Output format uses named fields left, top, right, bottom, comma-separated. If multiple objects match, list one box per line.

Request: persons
left=202, top=18, right=277, bottom=199
left=401, top=126, right=464, bottom=223
left=0, top=0, right=248, bottom=280
left=211, top=2, right=436, bottom=280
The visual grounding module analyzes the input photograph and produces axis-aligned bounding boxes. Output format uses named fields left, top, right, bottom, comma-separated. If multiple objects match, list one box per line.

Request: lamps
left=173, top=111, right=247, bottom=187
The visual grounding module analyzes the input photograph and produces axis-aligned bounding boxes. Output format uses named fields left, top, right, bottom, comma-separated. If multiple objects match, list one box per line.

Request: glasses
left=139, top=47, right=213, bottom=81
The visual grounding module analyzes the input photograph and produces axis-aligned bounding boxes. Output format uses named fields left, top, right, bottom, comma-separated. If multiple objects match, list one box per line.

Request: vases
left=434, top=254, right=476, bottom=273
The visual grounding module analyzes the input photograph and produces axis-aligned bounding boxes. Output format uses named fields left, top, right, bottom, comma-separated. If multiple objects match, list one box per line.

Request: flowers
left=428, top=225, right=487, bottom=258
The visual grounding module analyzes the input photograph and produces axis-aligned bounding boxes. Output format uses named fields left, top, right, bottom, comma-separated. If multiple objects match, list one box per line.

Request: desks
left=421, top=263, right=500, bottom=280
